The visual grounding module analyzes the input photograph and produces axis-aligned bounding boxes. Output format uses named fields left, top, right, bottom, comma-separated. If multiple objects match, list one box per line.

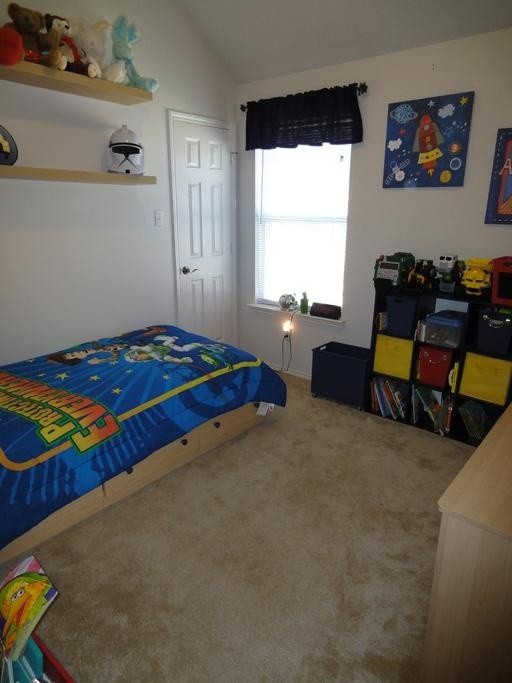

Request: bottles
left=300, top=292, right=309, bottom=314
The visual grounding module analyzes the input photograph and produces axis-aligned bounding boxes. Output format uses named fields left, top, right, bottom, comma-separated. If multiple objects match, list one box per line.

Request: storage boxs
left=477, top=308, right=512, bottom=355
left=487, top=255, right=512, bottom=306
left=425, top=310, right=465, bottom=349
left=386, top=294, right=418, bottom=337
left=311, top=341, right=370, bottom=411
left=420, top=346, right=452, bottom=387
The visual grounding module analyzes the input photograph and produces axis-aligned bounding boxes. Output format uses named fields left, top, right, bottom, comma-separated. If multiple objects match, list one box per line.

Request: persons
left=48, top=327, right=166, bottom=364
left=124, top=335, right=202, bottom=363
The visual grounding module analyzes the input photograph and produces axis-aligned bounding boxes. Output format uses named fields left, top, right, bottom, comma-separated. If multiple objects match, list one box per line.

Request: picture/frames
left=484, top=127, right=512, bottom=224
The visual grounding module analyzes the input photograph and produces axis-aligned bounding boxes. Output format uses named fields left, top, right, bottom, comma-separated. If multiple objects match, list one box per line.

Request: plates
left=0, top=124, right=18, bottom=165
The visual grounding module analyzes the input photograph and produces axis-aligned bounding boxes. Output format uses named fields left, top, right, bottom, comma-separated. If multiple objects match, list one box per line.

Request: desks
left=421, top=399, right=512, bottom=682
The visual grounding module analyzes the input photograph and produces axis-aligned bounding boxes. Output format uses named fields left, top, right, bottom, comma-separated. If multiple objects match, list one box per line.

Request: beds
left=0, top=325, right=287, bottom=564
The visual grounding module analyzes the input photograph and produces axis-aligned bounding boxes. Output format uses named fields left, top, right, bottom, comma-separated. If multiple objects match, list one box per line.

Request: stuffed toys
left=45, top=14, right=98, bottom=78
left=0, top=3, right=60, bottom=65
left=102, top=15, right=159, bottom=92
left=70, top=18, right=123, bottom=83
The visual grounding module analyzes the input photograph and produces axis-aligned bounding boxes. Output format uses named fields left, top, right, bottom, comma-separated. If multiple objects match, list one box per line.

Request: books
left=412, top=384, right=420, bottom=425
left=416, top=385, right=441, bottom=429
left=369, top=376, right=411, bottom=420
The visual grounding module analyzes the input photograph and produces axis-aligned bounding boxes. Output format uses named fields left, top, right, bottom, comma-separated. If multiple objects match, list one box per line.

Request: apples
left=441, top=272, right=454, bottom=285
left=457, top=260, right=465, bottom=272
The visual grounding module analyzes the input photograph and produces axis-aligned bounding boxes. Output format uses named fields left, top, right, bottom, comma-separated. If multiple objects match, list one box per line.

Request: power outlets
left=280, top=333, right=290, bottom=343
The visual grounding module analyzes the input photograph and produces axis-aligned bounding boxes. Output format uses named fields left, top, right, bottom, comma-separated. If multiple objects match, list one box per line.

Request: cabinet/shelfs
left=0, top=60, right=157, bottom=185
left=365, top=252, right=512, bottom=447
